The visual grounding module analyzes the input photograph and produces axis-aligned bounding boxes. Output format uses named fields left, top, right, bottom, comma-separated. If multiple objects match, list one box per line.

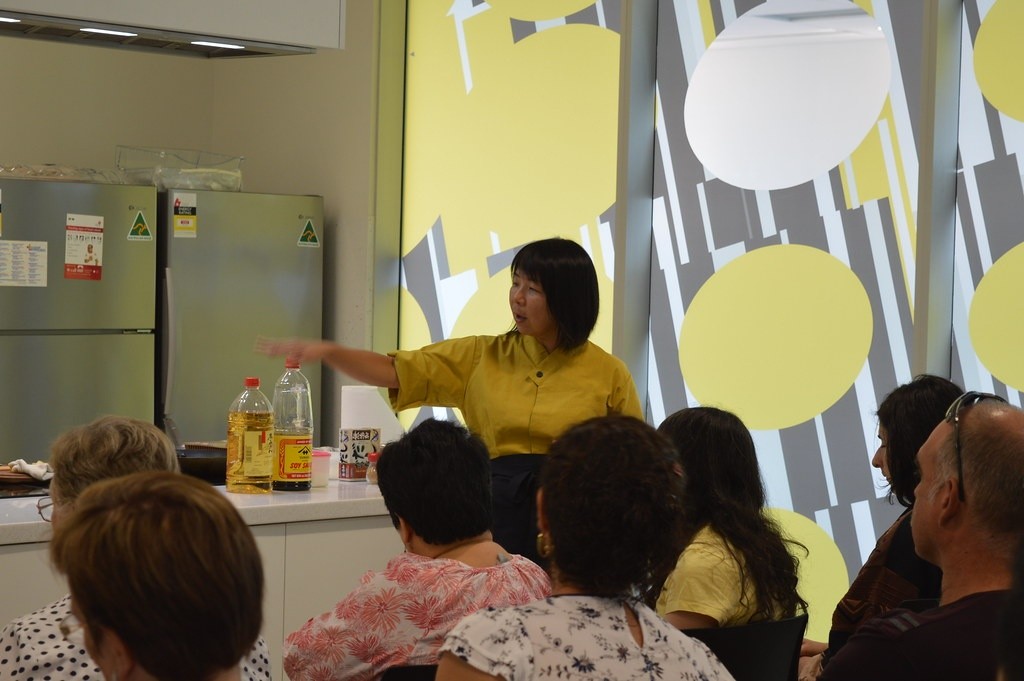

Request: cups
left=310, top=450, right=331, bottom=487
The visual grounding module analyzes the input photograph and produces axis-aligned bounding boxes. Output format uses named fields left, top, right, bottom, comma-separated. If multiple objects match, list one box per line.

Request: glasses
left=944, top=390, right=1009, bottom=501
left=36, top=496, right=64, bottom=522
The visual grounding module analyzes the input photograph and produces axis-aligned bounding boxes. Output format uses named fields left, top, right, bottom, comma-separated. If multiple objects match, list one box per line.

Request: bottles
left=272, top=357, right=314, bottom=490
left=224, top=377, right=275, bottom=495
left=365, top=452, right=381, bottom=484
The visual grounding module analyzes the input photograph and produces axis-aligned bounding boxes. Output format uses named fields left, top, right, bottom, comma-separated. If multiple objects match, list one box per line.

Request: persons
left=656, top=407, right=809, bottom=629
left=798, top=375, right=964, bottom=681
left=52, top=471, right=264, bottom=681
left=817, top=391, right=1024, bottom=681
left=434, top=413, right=739, bottom=681
left=282, top=419, right=555, bottom=681
left=255, top=239, right=647, bottom=577
left=0, top=415, right=277, bottom=681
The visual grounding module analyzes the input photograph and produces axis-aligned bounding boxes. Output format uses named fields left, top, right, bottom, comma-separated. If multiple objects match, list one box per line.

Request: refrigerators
left=161, top=186, right=324, bottom=446
left=0, top=177, right=157, bottom=468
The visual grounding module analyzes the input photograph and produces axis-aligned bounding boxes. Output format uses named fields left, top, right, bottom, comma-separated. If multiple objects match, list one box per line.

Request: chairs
left=676, top=610, right=811, bottom=681
